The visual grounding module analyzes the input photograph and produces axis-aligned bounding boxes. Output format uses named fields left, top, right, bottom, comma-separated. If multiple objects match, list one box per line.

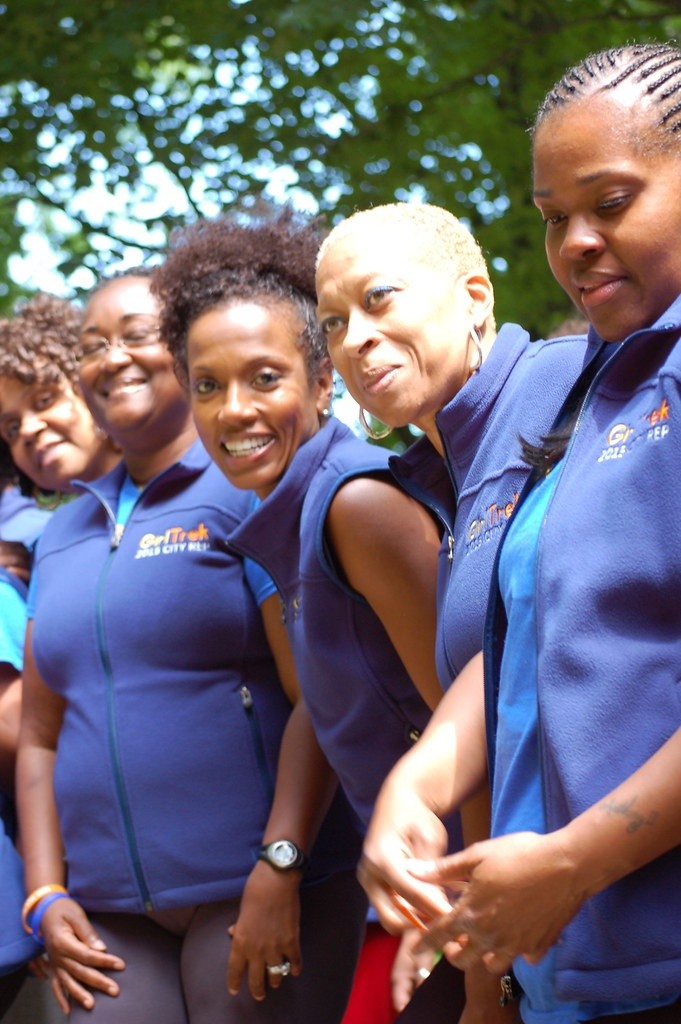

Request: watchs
left=255, top=838, right=316, bottom=874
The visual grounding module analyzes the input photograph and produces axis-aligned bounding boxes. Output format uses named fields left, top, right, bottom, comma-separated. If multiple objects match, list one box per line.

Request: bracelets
left=20, top=883, right=73, bottom=947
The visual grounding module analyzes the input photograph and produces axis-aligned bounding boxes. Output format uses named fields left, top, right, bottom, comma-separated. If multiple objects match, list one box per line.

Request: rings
left=266, top=962, right=291, bottom=976
left=415, top=967, right=430, bottom=978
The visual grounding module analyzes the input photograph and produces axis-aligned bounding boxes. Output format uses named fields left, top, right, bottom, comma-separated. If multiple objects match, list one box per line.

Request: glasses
left=73, top=324, right=163, bottom=361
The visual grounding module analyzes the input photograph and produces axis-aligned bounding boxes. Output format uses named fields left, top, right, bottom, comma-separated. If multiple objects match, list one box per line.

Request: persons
left=0, top=42, right=681, bottom=1023
